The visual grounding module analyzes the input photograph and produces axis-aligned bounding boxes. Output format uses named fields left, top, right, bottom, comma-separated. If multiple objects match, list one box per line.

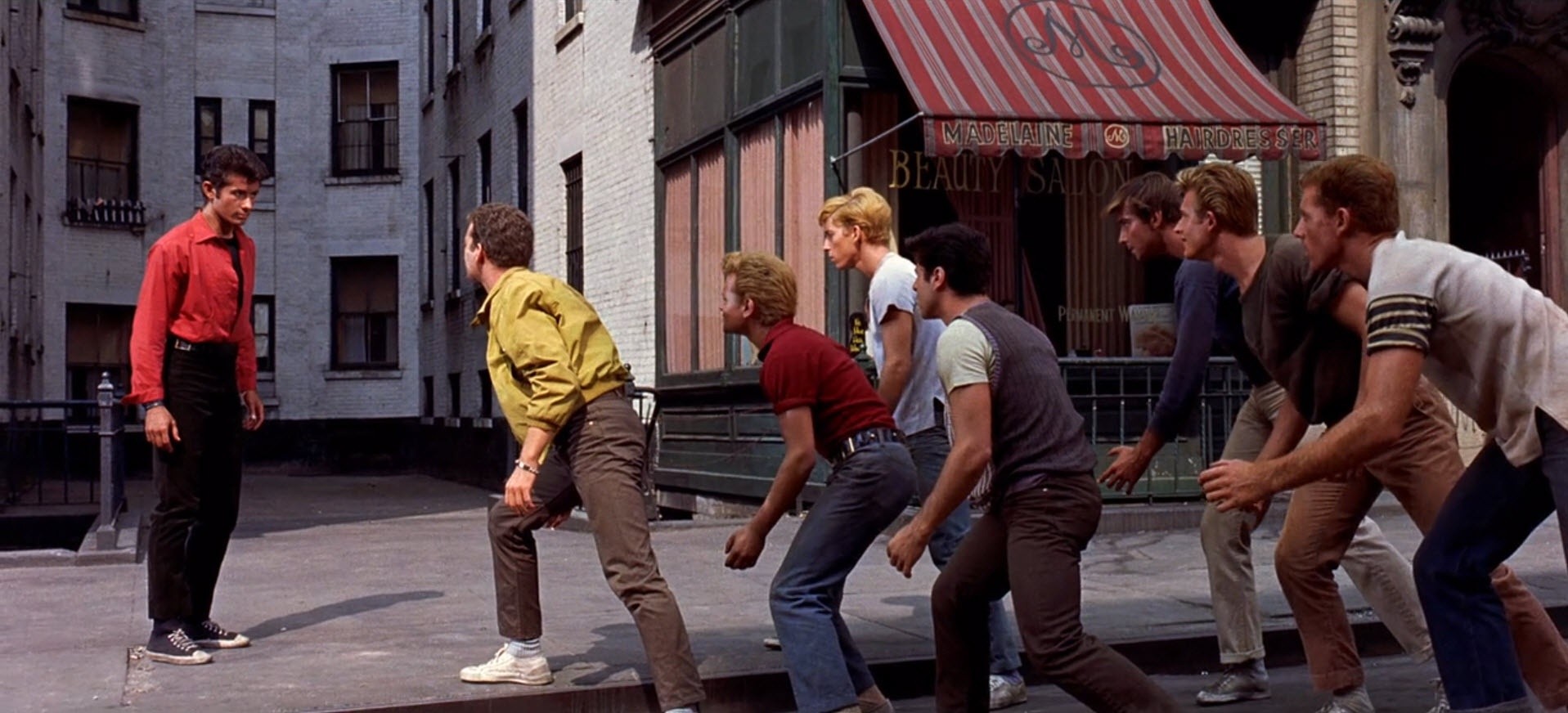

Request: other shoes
left=864, top=698, right=894, bottom=713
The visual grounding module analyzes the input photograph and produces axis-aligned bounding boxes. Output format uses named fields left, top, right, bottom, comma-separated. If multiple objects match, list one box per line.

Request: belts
left=833, top=428, right=908, bottom=466
left=173, top=338, right=196, bottom=352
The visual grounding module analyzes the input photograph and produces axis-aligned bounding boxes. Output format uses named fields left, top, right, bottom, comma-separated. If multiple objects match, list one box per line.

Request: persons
left=458, top=202, right=708, bottom=713
left=718, top=250, right=918, bottom=713
left=1173, top=159, right=1568, bottom=713
left=1198, top=153, right=1568, bottom=713
left=1096, top=172, right=1450, bottom=712
left=819, top=186, right=1026, bottom=709
left=885, top=222, right=1181, bottom=713
left=122, top=144, right=268, bottom=666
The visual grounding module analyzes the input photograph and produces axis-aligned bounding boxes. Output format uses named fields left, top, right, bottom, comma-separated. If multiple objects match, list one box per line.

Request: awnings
left=833, top=0, right=1320, bottom=163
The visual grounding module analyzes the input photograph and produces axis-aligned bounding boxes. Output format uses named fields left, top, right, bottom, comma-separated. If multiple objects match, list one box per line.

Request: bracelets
left=515, top=460, right=539, bottom=475
left=145, top=401, right=163, bottom=411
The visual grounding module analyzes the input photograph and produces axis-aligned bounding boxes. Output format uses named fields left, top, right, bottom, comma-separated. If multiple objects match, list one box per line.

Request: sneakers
left=1314, top=684, right=1376, bottom=713
left=181, top=618, right=250, bottom=648
left=763, top=636, right=781, bottom=649
left=146, top=627, right=212, bottom=665
left=460, top=640, right=553, bottom=686
left=1425, top=676, right=1450, bottom=713
left=1197, top=668, right=1271, bottom=702
left=988, top=673, right=1027, bottom=709
